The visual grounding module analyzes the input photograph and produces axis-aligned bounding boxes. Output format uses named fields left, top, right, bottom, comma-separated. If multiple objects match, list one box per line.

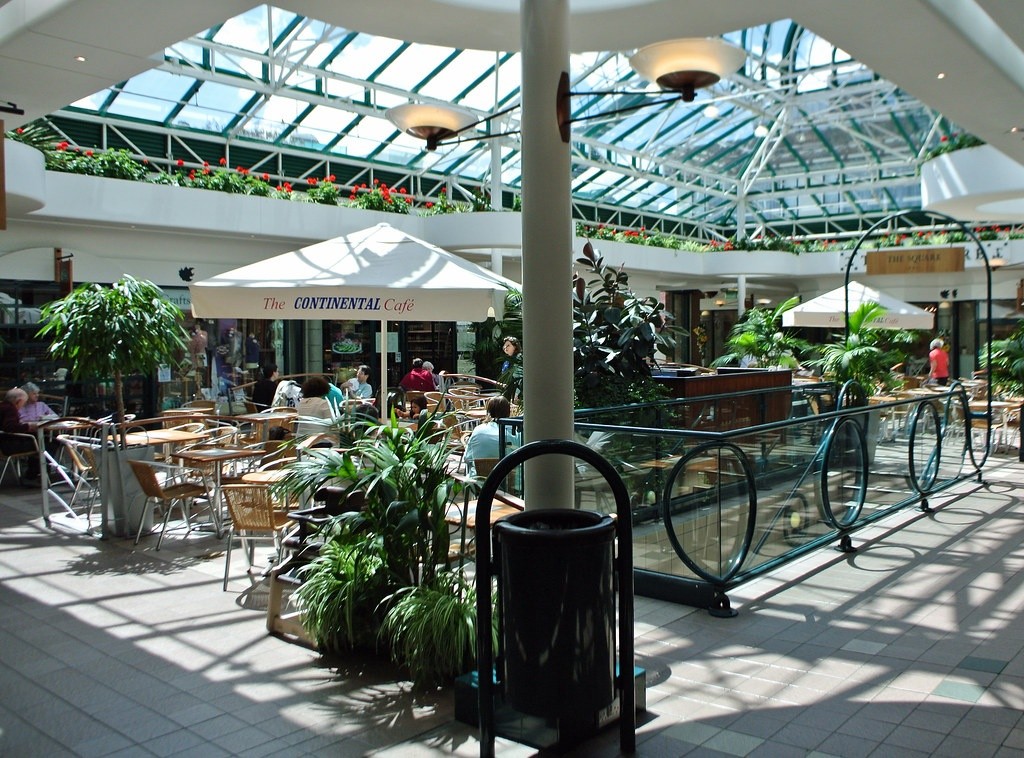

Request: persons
left=0, top=389, right=56, bottom=483
left=463, top=396, right=520, bottom=499
left=423, top=361, right=440, bottom=386
left=294, top=377, right=337, bottom=448
left=928, top=338, right=950, bottom=386
left=400, top=358, right=435, bottom=391
left=394, top=397, right=429, bottom=424
left=340, top=365, right=373, bottom=400
left=18, top=382, right=92, bottom=473
left=252, top=364, right=278, bottom=413
left=502, top=336, right=522, bottom=376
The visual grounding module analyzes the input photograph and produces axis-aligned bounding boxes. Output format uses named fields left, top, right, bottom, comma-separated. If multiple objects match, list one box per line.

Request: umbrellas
left=782, top=281, right=935, bottom=329
left=188, top=222, right=521, bottom=426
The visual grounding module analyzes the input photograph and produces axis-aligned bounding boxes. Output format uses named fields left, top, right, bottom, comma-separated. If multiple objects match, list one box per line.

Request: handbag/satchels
left=269, top=426, right=290, bottom=440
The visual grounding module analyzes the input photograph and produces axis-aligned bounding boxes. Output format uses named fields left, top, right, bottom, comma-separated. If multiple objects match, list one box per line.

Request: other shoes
left=78, top=465, right=93, bottom=473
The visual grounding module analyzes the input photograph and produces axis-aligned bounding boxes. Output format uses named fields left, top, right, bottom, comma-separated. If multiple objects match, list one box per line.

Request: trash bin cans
left=491, top=508, right=616, bottom=719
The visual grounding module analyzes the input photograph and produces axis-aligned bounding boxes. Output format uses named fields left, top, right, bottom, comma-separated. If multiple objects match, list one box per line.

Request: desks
left=241, top=471, right=300, bottom=555
left=106, top=429, right=210, bottom=517
left=970, top=399, right=1022, bottom=453
left=235, top=413, right=299, bottom=441
left=29, top=420, right=93, bottom=488
left=170, top=449, right=266, bottom=537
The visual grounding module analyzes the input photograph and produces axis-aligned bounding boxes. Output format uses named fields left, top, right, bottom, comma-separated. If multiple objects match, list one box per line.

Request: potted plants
left=812, top=303, right=918, bottom=466
left=265, top=417, right=499, bottom=678
left=38, top=274, right=190, bottom=537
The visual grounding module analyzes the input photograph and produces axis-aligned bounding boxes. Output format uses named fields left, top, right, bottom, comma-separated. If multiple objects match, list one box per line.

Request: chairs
left=868, top=376, right=1024, bottom=457
left=0, top=380, right=523, bottom=593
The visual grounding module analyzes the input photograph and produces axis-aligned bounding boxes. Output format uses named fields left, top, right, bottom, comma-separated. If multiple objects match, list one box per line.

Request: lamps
left=629, top=37, right=746, bottom=103
left=386, top=104, right=480, bottom=150
